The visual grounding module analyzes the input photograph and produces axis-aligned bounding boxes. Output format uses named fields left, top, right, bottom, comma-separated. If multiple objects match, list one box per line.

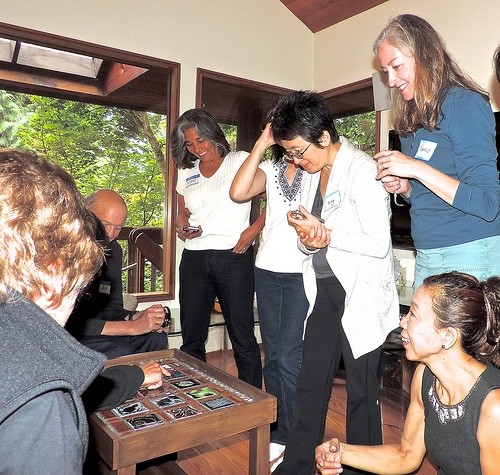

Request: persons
left=372, top=13, right=499, bottom=291
left=169, top=107, right=267, bottom=390
left=313, top=270, right=499, bottom=475
left=79, top=358, right=172, bottom=414
left=228, top=117, right=305, bottom=463
left=269, top=91, right=401, bottom=475
left=63, top=189, right=169, bottom=360
left=0, top=149, right=110, bottom=475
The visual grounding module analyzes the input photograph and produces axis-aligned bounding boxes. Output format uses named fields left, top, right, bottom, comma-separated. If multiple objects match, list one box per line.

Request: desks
left=89, top=348, right=280, bottom=475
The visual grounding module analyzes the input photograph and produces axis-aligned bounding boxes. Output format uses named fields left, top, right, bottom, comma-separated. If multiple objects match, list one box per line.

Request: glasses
left=285, top=142, right=313, bottom=159
